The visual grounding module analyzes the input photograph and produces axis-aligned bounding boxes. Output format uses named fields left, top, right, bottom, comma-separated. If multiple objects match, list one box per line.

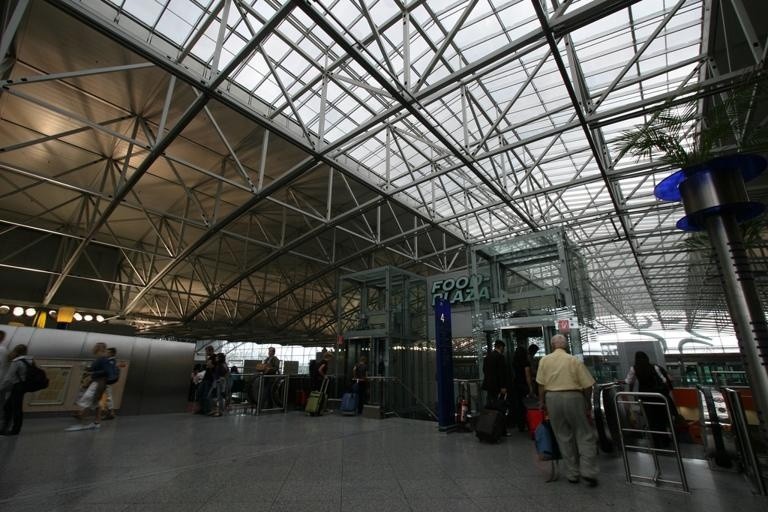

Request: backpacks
left=15, top=359, right=48, bottom=393
left=106, top=359, right=119, bottom=385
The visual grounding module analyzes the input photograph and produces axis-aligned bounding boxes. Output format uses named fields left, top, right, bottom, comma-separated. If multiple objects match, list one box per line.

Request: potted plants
left=613, top=57, right=768, bottom=220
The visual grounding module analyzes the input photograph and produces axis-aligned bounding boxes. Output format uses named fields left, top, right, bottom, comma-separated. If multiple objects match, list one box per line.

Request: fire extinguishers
left=456, top=394, right=469, bottom=422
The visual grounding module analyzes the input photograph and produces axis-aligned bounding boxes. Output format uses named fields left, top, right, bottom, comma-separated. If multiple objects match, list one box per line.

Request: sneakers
left=506, top=432, right=512, bottom=436
left=101, top=413, right=115, bottom=420
left=64, top=422, right=100, bottom=431
left=568, top=476, right=598, bottom=487
left=0, top=427, right=19, bottom=435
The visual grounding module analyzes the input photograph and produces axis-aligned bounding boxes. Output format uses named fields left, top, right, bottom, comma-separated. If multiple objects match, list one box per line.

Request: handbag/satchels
left=534, top=420, right=562, bottom=460
left=672, top=413, right=689, bottom=436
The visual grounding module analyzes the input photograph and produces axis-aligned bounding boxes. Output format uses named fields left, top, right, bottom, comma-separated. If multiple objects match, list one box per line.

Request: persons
left=208, top=354, right=230, bottom=417
left=256, top=347, right=280, bottom=409
left=617, top=350, right=679, bottom=454
left=229, top=367, right=242, bottom=403
left=535, top=333, right=599, bottom=484
left=314, top=352, right=334, bottom=413
left=483, top=338, right=513, bottom=438
left=513, top=344, right=533, bottom=434
left=193, top=344, right=218, bottom=413
left=528, top=341, right=539, bottom=370
left=0, top=344, right=35, bottom=436
left=0, top=329, right=12, bottom=401
left=353, top=356, right=369, bottom=413
left=99, top=347, right=120, bottom=418
left=72, top=341, right=110, bottom=420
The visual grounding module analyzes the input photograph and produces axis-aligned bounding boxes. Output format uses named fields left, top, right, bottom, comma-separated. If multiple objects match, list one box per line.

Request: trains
left=582, top=349, right=752, bottom=389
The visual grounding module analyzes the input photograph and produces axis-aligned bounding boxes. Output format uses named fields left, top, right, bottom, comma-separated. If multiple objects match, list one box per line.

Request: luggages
left=476, top=391, right=508, bottom=443
left=525, top=394, right=546, bottom=440
left=305, top=377, right=330, bottom=416
left=340, top=378, right=360, bottom=416
